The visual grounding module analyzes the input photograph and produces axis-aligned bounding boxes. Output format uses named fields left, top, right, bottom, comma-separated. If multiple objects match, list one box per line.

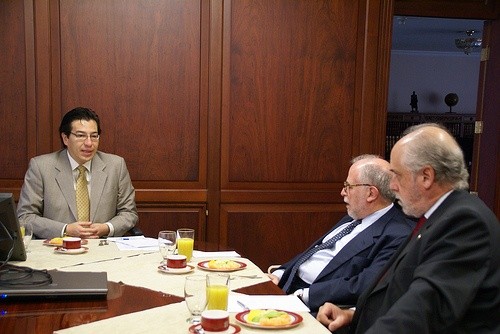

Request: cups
left=167, top=254, right=187, bottom=268
left=20, top=221, right=33, bottom=250
left=177, top=228, right=195, bottom=261
left=158, top=231, right=176, bottom=259
left=63, top=237, right=81, bottom=249
left=201, top=313, right=230, bottom=332
left=206, top=272, right=230, bottom=312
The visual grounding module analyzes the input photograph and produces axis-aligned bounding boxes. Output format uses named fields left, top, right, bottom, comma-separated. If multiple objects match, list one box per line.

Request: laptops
left=0, top=270, right=108, bottom=297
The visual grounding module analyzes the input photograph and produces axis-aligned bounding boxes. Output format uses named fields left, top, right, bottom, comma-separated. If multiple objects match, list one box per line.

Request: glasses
left=69, top=130, right=100, bottom=142
left=343, top=180, right=380, bottom=195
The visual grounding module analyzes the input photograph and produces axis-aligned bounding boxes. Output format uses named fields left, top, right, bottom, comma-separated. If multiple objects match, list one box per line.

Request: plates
left=197, top=259, right=246, bottom=271
left=44, top=237, right=89, bottom=246
left=235, top=309, right=303, bottom=329
left=55, top=248, right=89, bottom=254
left=158, top=264, right=195, bottom=273
left=187, top=323, right=241, bottom=334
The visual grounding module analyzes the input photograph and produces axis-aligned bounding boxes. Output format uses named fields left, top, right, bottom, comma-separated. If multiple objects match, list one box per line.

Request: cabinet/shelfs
left=385, top=112, right=475, bottom=179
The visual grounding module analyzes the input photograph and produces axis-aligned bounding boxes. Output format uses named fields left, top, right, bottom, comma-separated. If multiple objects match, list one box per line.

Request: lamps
left=455, top=29, right=482, bottom=56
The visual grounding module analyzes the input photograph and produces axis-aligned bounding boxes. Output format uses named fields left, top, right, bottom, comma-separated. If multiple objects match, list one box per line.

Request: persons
left=314, top=122, right=500, bottom=334
left=264, top=153, right=414, bottom=317
left=17, top=107, right=139, bottom=239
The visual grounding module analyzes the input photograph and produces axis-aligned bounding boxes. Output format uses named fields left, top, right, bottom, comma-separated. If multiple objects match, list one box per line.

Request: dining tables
left=0, top=238, right=333, bottom=334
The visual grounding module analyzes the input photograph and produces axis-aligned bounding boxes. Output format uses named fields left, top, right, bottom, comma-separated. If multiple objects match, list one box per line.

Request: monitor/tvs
left=0, top=193, right=27, bottom=263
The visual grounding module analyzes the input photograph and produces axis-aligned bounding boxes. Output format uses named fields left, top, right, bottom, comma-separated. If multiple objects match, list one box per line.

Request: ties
left=282, top=219, right=363, bottom=294
left=412, top=215, right=427, bottom=237
left=75, top=165, right=90, bottom=229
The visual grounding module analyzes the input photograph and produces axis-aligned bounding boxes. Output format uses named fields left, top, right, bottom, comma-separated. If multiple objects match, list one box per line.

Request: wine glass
left=184, top=275, right=208, bottom=325
